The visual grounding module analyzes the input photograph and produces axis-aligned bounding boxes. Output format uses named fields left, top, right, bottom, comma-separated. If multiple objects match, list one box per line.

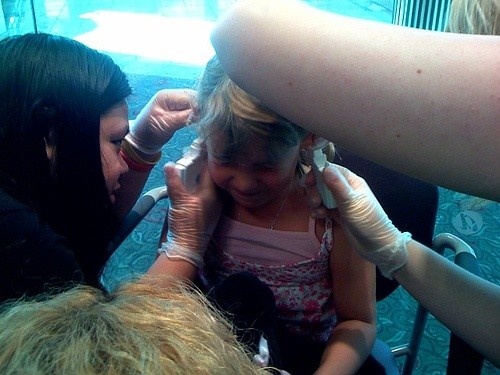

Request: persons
left=210, top=0, right=500, bottom=375
left=196, top=52, right=376, bottom=375
left=0, top=279, right=272, bottom=375
left=0, top=33, right=222, bottom=309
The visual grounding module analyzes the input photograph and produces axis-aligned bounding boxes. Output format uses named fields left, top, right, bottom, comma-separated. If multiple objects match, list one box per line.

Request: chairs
left=107, top=142, right=484, bottom=375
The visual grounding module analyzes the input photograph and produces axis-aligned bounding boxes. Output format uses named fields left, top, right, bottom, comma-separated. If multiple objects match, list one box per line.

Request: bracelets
left=119, top=141, right=161, bottom=173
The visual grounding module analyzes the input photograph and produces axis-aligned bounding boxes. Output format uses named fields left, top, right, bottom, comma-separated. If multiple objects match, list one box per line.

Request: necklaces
left=235, top=188, right=290, bottom=231
